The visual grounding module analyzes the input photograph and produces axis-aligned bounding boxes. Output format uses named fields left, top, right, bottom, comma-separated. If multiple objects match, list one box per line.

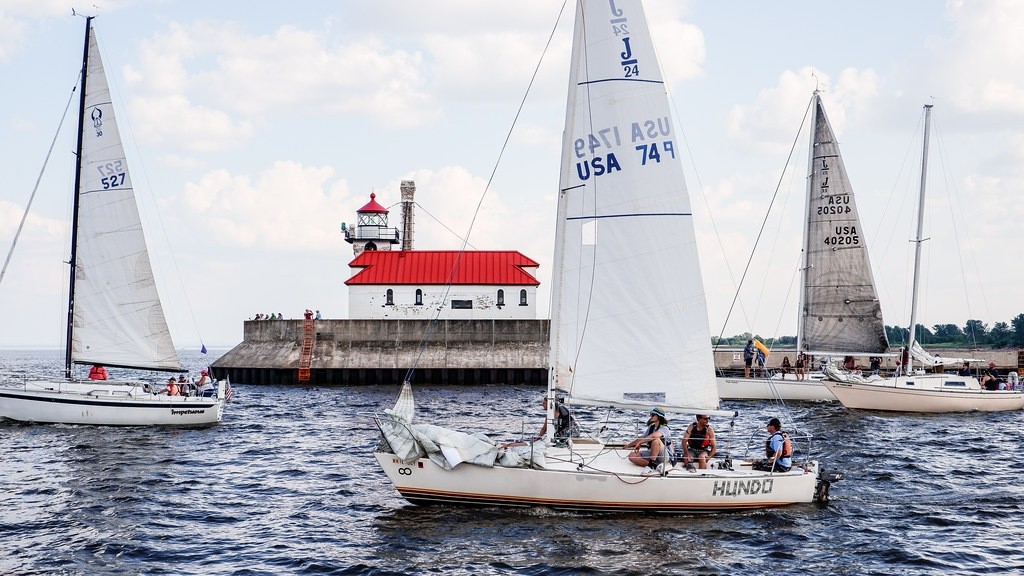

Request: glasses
left=652, top=413, right=657, bottom=417
left=704, top=417, right=710, bottom=420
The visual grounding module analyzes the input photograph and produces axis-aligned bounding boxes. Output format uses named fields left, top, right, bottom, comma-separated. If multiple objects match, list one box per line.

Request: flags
left=201, top=345, right=208, bottom=354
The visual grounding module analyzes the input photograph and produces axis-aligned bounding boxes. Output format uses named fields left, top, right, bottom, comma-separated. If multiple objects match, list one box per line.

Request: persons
left=255, top=313, right=283, bottom=320
left=869, top=357, right=883, bottom=375
left=980, top=362, right=1011, bottom=391
left=781, top=357, right=790, bottom=379
left=932, top=354, right=944, bottom=373
left=681, top=414, right=716, bottom=470
left=195, top=371, right=216, bottom=397
left=743, top=340, right=756, bottom=379
left=160, top=373, right=192, bottom=397
left=800, top=350, right=815, bottom=370
left=755, top=347, right=766, bottom=378
left=533, top=396, right=580, bottom=447
left=795, top=355, right=805, bottom=381
left=88, top=364, right=108, bottom=380
left=624, top=407, right=677, bottom=475
left=752, top=418, right=792, bottom=472
left=304, top=309, right=322, bottom=320
left=843, top=355, right=863, bottom=377
left=959, top=361, right=970, bottom=376
left=894, top=346, right=908, bottom=377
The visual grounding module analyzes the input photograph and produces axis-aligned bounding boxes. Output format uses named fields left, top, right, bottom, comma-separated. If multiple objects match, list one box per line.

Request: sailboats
left=0, top=8, right=231, bottom=431
left=713, top=70, right=1024, bottom=418
left=368, top=1, right=837, bottom=520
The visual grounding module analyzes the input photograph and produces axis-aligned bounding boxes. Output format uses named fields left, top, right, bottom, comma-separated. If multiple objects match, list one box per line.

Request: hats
left=201, top=369, right=208, bottom=374
left=652, top=407, right=665, bottom=417
left=180, top=374, right=185, bottom=378
